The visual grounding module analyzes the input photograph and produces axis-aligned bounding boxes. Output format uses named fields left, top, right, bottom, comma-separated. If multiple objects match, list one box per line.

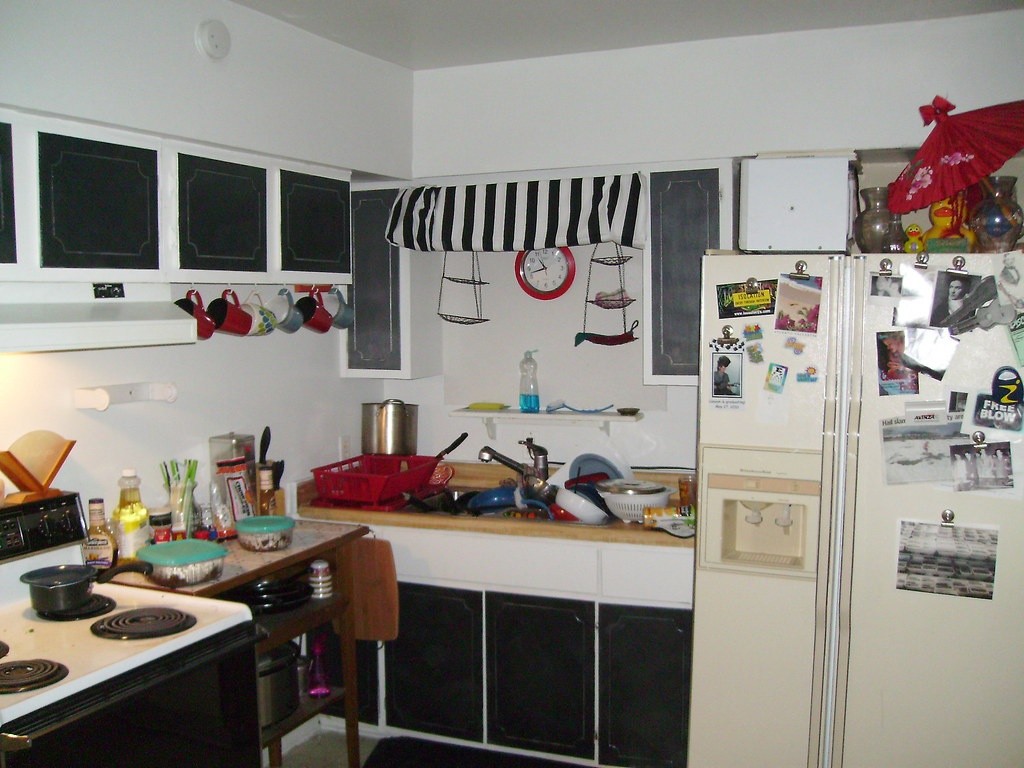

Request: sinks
left=398, top=486, right=503, bottom=518
left=503, top=489, right=619, bottom=527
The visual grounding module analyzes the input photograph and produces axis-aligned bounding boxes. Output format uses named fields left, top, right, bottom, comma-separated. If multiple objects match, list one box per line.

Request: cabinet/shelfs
left=314, top=517, right=694, bottom=767
left=642, top=159, right=740, bottom=386
left=111, top=520, right=404, bottom=768
left=0, top=118, right=355, bottom=283
left=340, top=179, right=439, bottom=379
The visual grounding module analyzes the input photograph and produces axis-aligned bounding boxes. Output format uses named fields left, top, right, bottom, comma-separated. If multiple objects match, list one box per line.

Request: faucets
left=478, top=440, right=549, bottom=481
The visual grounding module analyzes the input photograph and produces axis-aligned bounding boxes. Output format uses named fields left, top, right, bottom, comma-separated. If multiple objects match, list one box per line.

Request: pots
left=20, top=560, right=153, bottom=614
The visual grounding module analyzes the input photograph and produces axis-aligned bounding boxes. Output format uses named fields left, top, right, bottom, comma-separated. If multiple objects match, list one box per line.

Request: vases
left=881, top=211, right=908, bottom=252
left=855, top=187, right=892, bottom=254
left=970, top=176, right=1024, bottom=253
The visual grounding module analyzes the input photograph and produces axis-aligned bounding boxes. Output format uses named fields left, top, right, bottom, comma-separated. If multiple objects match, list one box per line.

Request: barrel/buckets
left=362, top=399, right=418, bottom=456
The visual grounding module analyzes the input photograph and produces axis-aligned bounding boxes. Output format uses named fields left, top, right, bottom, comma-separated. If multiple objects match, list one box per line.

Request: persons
left=933, top=275, right=974, bottom=328
left=879, top=333, right=919, bottom=382
left=996, top=253, right=1023, bottom=306
left=953, top=449, right=1015, bottom=490
left=712, top=356, right=737, bottom=396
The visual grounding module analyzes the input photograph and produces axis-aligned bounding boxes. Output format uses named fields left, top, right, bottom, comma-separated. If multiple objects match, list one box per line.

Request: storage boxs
left=138, top=538, right=229, bottom=587
left=235, top=517, right=297, bottom=551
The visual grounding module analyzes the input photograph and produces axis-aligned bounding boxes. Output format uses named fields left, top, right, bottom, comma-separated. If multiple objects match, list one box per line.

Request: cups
left=174, top=287, right=355, bottom=340
left=309, top=559, right=332, bottom=600
left=678, top=475, right=697, bottom=505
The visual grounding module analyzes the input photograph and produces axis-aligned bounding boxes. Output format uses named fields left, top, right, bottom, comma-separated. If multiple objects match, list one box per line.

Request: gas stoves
left=0, top=490, right=254, bottom=731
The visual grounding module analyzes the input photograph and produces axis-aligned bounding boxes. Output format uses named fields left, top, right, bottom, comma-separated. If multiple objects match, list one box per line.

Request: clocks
left=515, top=246, right=577, bottom=301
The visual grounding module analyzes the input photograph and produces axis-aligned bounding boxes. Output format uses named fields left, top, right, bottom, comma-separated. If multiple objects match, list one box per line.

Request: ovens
left=0, top=621, right=263, bottom=767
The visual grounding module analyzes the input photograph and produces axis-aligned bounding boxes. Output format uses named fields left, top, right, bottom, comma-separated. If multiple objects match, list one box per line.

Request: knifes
left=259, top=425, right=271, bottom=465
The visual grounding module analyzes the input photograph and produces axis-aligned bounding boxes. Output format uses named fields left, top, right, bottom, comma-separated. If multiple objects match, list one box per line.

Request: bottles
left=307, top=633, right=330, bottom=700
left=853, top=185, right=891, bottom=253
left=519, top=349, right=540, bottom=414
left=968, top=176, right=1023, bottom=254
left=111, top=469, right=150, bottom=569
left=80, top=497, right=120, bottom=579
left=258, top=466, right=276, bottom=515
left=643, top=503, right=696, bottom=530
left=881, top=212, right=909, bottom=253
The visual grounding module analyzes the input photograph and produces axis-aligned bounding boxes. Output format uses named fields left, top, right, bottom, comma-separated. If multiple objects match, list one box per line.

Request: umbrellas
left=888, top=96, right=1024, bottom=238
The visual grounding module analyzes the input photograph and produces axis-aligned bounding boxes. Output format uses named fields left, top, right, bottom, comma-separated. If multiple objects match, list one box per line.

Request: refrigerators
left=683, top=246, right=1024, bottom=768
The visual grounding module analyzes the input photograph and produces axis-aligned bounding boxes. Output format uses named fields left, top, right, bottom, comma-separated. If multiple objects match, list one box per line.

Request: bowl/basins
left=136, top=539, right=229, bottom=589
left=233, top=516, right=295, bottom=552
left=616, top=408, right=640, bottom=416
left=466, top=451, right=624, bottom=525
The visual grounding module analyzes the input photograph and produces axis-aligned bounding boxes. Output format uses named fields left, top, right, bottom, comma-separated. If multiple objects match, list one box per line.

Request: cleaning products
left=519, top=350, right=541, bottom=414
